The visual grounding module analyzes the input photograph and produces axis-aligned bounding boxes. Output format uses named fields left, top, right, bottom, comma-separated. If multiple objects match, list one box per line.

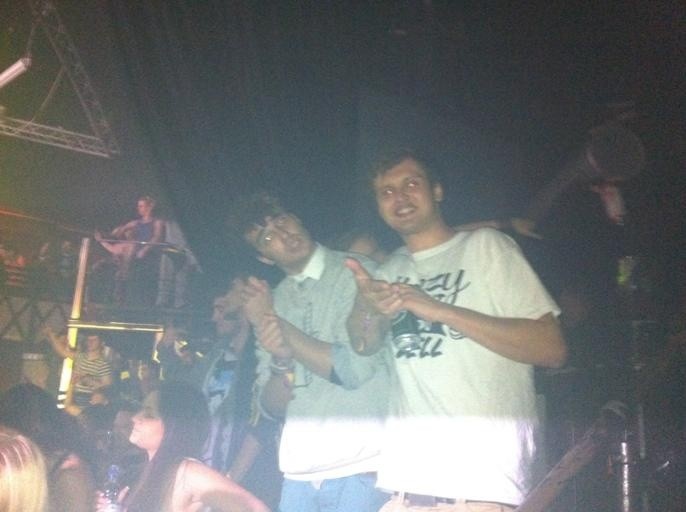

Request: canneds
left=390, top=309, right=421, bottom=351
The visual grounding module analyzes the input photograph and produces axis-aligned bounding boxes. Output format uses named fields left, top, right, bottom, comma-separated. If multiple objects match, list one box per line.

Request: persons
left=0, top=97, right=686, bottom=512
